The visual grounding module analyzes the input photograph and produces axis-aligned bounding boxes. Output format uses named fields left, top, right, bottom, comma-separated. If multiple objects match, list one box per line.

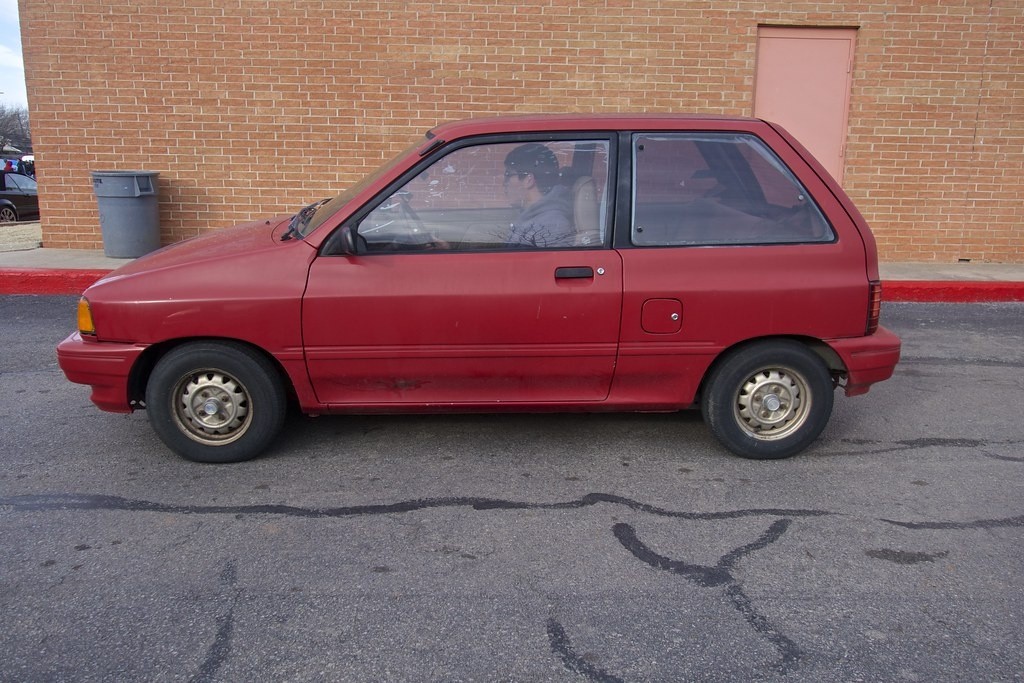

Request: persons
left=4, top=161, right=14, bottom=172
left=430, top=142, right=574, bottom=248
left=16, top=159, right=36, bottom=179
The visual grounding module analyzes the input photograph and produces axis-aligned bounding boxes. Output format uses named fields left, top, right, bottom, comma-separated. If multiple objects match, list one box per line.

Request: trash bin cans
left=91, top=170, right=162, bottom=260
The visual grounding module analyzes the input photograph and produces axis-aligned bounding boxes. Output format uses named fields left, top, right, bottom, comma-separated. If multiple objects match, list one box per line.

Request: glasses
left=504, top=171, right=530, bottom=178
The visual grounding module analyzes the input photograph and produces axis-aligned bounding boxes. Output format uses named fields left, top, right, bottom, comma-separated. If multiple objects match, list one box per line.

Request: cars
left=0, top=159, right=25, bottom=173
left=0, top=169, right=40, bottom=223
left=53, top=110, right=903, bottom=465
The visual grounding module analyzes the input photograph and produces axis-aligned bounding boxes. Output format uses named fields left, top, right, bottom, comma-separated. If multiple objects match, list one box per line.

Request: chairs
left=560, top=166, right=599, bottom=245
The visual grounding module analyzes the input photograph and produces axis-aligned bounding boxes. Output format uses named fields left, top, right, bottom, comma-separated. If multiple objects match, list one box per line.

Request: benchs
left=648, top=197, right=805, bottom=242
